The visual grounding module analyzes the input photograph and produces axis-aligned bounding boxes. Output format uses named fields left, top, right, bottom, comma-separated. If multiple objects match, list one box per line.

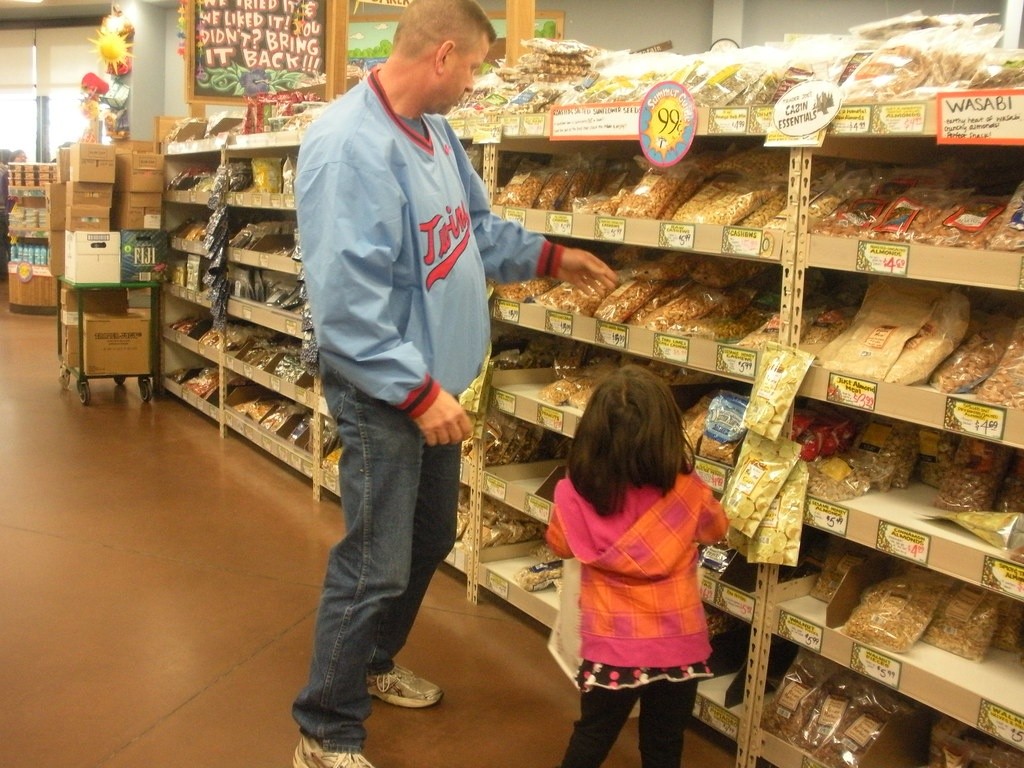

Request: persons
left=292, top=0, right=619, bottom=767
left=543, top=365, right=731, bottom=767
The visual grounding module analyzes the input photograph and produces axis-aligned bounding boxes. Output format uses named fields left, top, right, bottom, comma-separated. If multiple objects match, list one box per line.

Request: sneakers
left=366, top=663, right=444, bottom=708
left=293, top=735, right=375, bottom=768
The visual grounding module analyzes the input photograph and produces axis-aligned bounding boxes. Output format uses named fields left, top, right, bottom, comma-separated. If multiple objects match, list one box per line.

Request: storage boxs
left=45, top=138, right=165, bottom=376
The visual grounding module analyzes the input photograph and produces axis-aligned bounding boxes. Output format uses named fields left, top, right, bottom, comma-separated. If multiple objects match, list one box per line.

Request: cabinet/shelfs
left=7, top=160, right=60, bottom=314
left=157, top=86, right=1023, bottom=768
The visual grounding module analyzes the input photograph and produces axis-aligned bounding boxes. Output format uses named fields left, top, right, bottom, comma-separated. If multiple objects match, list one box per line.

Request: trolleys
left=53, top=275, right=162, bottom=404
